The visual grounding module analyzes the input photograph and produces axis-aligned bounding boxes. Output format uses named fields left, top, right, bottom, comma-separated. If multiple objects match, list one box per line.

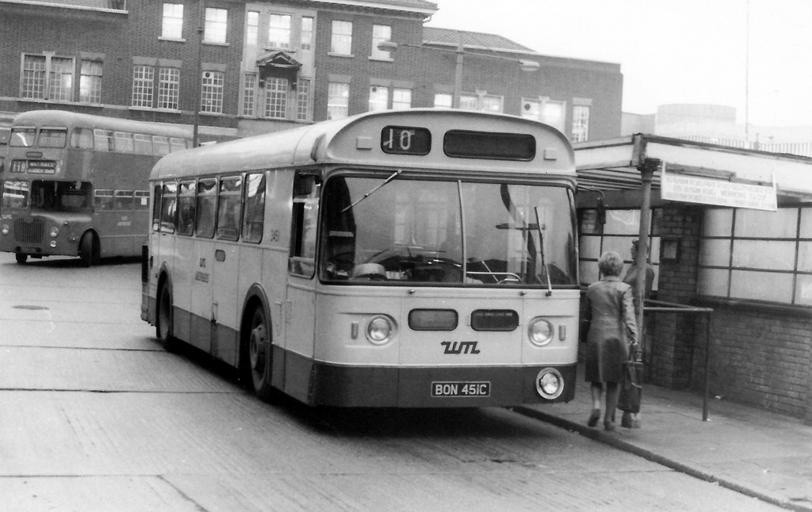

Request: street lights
left=377, top=34, right=541, bottom=108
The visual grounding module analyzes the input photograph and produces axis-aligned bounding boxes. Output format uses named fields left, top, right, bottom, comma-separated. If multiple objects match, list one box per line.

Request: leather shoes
left=603, top=420, right=615, bottom=431
left=587, top=408, right=601, bottom=427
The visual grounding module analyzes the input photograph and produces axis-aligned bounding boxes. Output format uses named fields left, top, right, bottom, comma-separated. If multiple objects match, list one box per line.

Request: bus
left=1, top=110, right=201, bottom=268
left=141, top=107, right=582, bottom=408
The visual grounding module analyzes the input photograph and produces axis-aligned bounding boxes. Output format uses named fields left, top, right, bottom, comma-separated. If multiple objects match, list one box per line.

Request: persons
left=580, top=249, right=638, bottom=430
left=622, top=240, right=655, bottom=299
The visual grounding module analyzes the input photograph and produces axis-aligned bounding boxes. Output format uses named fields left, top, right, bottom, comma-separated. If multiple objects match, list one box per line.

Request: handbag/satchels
left=616, top=348, right=643, bottom=413
